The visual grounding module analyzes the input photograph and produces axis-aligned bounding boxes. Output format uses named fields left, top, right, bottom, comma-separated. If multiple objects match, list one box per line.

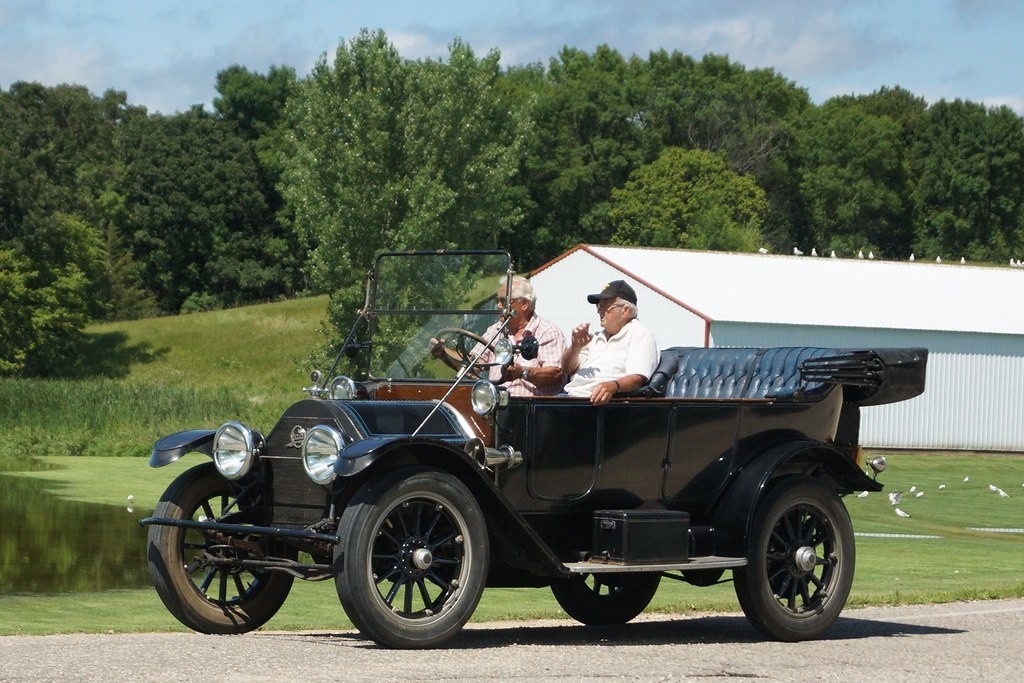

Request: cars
left=138, top=249, right=928, bottom=649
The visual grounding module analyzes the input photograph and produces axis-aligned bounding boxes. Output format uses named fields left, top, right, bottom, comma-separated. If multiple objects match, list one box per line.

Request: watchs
left=520, top=366, right=528, bottom=380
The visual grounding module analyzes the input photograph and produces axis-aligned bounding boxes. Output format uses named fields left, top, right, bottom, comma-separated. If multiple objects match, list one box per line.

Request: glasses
left=597, top=302, right=623, bottom=311
left=496, top=296, right=524, bottom=307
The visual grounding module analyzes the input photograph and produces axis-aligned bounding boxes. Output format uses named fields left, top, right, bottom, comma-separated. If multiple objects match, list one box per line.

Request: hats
left=587, top=280, right=638, bottom=306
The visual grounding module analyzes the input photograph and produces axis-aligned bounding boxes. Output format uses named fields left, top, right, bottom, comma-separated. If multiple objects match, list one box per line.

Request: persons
left=430, top=274, right=569, bottom=398
left=561, top=280, right=658, bottom=407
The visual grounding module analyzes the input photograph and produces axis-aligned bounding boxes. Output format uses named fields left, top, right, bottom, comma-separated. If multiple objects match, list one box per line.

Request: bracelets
left=613, top=380, right=620, bottom=392
